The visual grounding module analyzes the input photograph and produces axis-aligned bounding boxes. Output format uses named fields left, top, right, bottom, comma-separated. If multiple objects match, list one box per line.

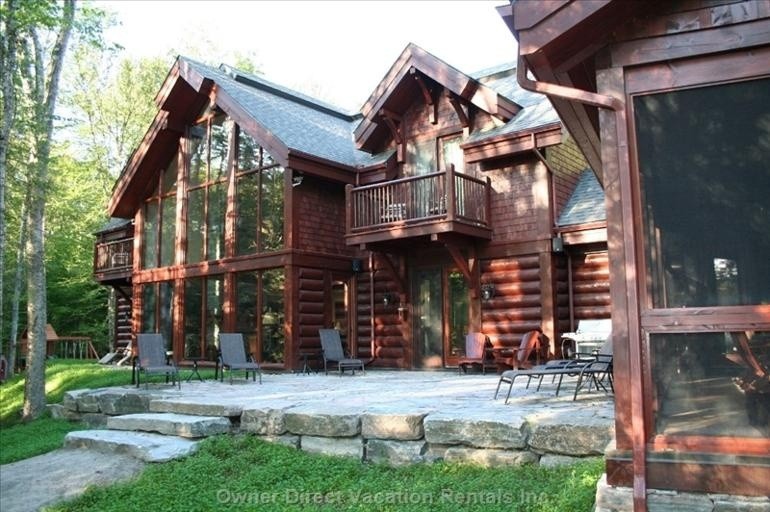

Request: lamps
left=381, top=290, right=398, bottom=312
left=478, top=280, right=496, bottom=302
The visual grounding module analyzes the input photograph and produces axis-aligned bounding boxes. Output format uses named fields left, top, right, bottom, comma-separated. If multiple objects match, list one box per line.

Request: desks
left=295, top=352, right=317, bottom=376
left=184, top=357, right=207, bottom=383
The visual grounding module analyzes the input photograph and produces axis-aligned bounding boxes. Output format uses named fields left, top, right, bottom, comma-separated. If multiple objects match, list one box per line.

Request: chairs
left=111, top=253, right=128, bottom=267
left=730, top=331, right=770, bottom=425
left=317, top=329, right=364, bottom=377
left=216, top=333, right=262, bottom=385
left=429, top=194, right=449, bottom=215
left=458, top=331, right=614, bottom=404
left=380, top=203, right=406, bottom=223
left=133, top=333, right=180, bottom=390
